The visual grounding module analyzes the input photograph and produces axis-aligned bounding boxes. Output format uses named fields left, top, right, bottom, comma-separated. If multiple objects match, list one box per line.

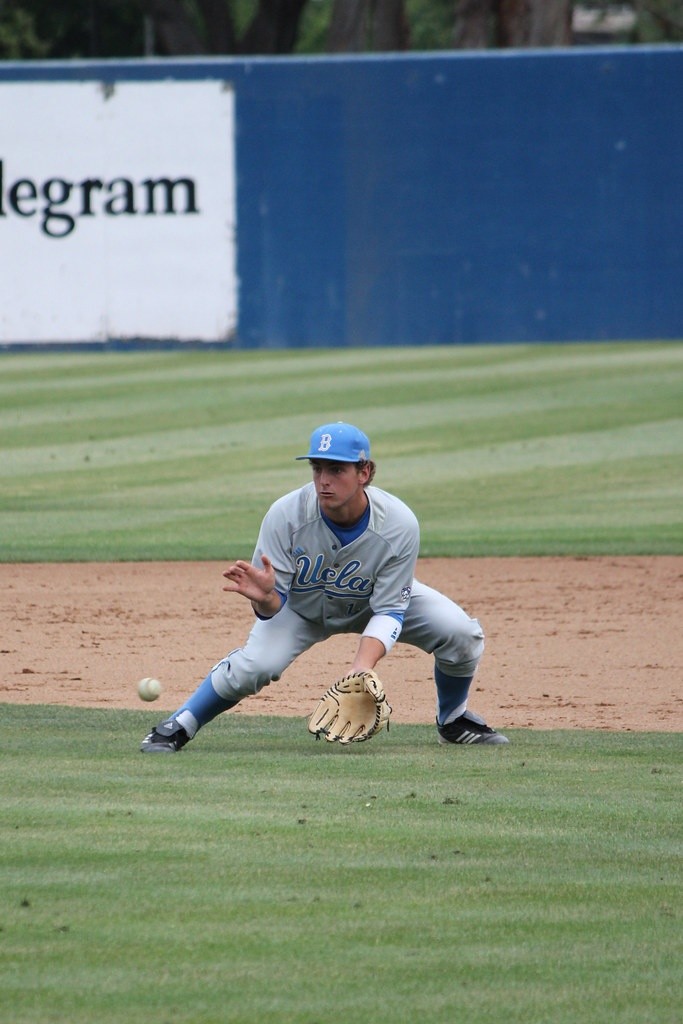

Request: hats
left=296, top=421, right=369, bottom=463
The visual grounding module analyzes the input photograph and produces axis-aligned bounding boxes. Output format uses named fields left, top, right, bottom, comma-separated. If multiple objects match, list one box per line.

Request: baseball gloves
left=305, top=667, right=393, bottom=748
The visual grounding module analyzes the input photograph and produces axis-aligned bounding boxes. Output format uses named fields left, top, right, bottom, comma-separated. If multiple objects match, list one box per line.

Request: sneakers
left=437, top=711, right=510, bottom=745
left=140, top=720, right=190, bottom=755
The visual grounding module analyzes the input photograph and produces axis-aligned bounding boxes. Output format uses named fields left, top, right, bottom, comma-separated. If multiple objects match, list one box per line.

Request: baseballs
left=137, top=677, right=162, bottom=702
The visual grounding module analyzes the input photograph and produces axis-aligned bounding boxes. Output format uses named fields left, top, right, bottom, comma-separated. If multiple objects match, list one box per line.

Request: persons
left=141, top=421, right=512, bottom=755
